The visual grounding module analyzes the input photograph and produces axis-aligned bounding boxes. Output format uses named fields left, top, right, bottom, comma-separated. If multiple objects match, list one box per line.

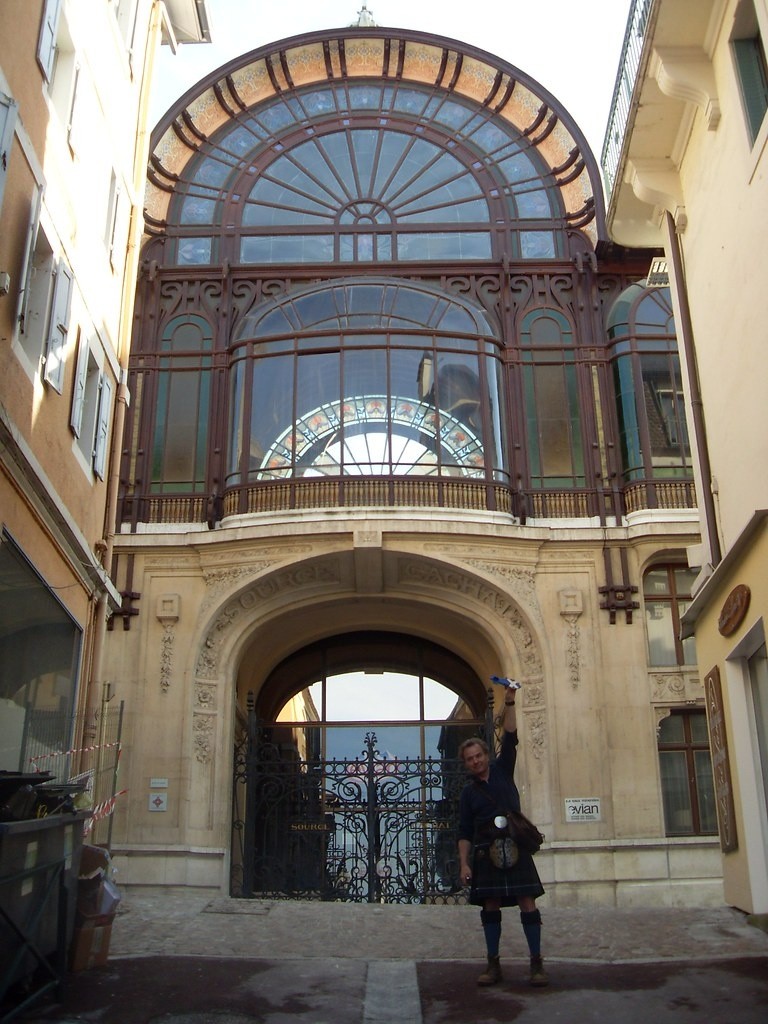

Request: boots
left=476, top=955, right=504, bottom=985
left=529, top=954, right=548, bottom=985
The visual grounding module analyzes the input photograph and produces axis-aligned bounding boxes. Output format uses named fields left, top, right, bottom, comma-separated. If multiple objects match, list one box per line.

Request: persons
left=456, top=679, right=554, bottom=984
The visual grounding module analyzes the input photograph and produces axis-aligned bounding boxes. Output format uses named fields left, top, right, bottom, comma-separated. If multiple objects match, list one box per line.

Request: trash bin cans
left=0, top=811, right=92, bottom=1024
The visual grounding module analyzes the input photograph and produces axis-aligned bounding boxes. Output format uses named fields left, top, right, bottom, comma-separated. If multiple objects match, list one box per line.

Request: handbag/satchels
left=507, top=808, right=543, bottom=855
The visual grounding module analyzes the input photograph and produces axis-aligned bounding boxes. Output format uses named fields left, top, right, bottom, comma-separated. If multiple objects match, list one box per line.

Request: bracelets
left=504, top=699, right=515, bottom=706
